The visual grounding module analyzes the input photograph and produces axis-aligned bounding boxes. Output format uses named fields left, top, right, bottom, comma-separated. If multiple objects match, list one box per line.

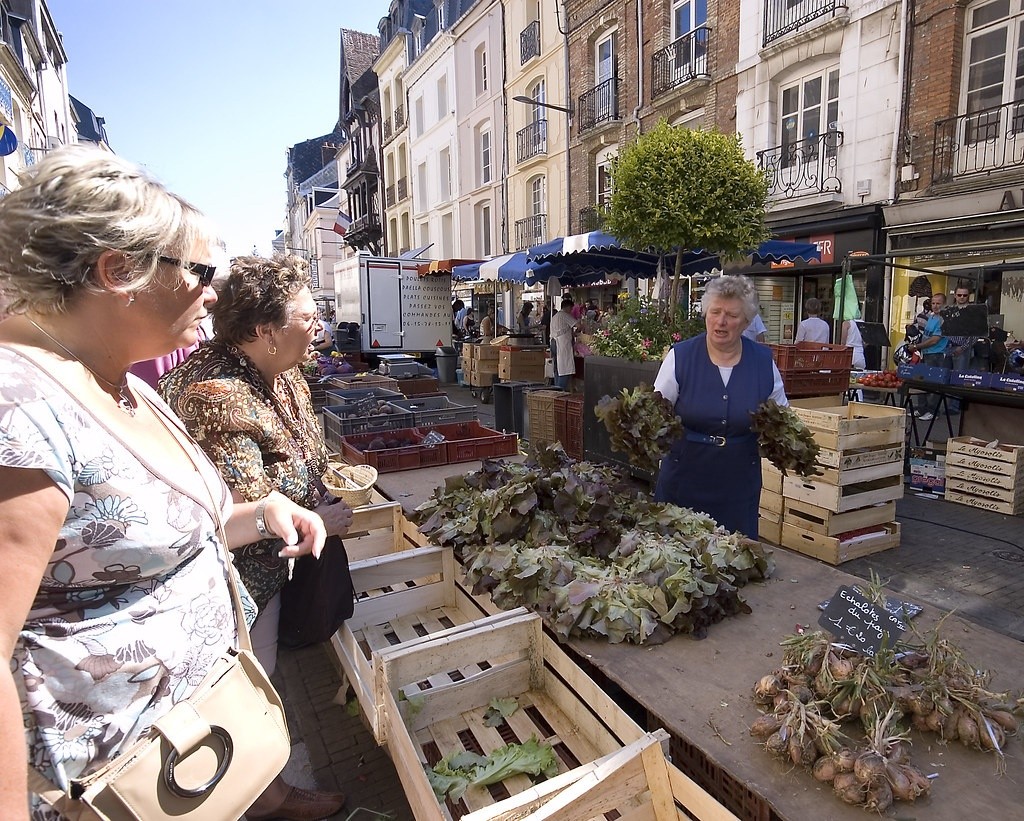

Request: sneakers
left=907, top=410, right=920, bottom=417
left=919, top=411, right=939, bottom=421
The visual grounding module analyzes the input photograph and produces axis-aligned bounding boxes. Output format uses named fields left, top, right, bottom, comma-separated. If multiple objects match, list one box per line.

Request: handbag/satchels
left=64, top=646, right=291, bottom=821
left=893, top=344, right=913, bottom=366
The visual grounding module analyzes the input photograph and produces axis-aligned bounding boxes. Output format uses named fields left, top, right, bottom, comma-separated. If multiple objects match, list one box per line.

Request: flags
left=333, top=213, right=351, bottom=235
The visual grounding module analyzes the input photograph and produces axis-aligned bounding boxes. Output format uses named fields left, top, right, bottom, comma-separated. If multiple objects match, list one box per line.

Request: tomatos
left=858, top=370, right=904, bottom=387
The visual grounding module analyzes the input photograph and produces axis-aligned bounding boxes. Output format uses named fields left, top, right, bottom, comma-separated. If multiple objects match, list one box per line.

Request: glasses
left=288, top=312, right=319, bottom=325
left=956, top=294, right=967, bottom=297
left=147, top=253, right=215, bottom=287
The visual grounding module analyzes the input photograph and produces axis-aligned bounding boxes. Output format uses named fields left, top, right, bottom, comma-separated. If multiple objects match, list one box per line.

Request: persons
left=794, top=298, right=830, bottom=359
left=518, top=293, right=604, bottom=388
left=906, top=284, right=976, bottom=420
left=651, top=272, right=791, bottom=541
left=741, top=314, right=767, bottom=343
left=842, top=319, right=865, bottom=402
left=1, top=142, right=352, bottom=821
left=451, top=299, right=507, bottom=350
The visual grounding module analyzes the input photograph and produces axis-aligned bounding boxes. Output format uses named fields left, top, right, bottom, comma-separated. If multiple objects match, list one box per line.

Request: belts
left=684, top=430, right=759, bottom=447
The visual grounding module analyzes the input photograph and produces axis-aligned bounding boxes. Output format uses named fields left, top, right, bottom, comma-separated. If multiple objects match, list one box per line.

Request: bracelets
left=914, top=344, right=917, bottom=350
left=254, top=497, right=278, bottom=539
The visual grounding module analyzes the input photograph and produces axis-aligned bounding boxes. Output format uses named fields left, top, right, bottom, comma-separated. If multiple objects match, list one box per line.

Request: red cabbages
left=316, top=356, right=353, bottom=375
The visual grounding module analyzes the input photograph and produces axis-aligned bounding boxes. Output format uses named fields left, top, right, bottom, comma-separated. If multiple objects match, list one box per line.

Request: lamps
left=512, top=96, right=575, bottom=118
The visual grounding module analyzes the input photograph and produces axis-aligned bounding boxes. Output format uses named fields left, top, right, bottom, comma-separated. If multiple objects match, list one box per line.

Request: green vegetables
left=749, top=399, right=824, bottom=477
left=423, top=697, right=558, bottom=804
left=594, top=381, right=681, bottom=469
left=414, top=441, right=774, bottom=646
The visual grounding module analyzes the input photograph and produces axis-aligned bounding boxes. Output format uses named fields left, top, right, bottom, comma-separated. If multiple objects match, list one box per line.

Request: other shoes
left=943, top=409, right=959, bottom=415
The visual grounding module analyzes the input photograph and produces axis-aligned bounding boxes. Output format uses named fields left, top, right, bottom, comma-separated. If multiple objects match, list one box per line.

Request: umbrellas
left=527, top=224, right=818, bottom=320
left=418, top=248, right=623, bottom=338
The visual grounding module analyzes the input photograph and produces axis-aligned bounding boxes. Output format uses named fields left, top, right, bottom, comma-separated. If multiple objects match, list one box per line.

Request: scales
left=377, top=352, right=439, bottom=379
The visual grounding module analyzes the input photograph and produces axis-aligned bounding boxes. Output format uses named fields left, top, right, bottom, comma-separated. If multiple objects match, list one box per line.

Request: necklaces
left=22, top=313, right=134, bottom=416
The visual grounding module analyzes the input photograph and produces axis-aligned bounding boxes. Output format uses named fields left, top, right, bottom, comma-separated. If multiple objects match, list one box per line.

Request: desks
left=906, top=379, right=1024, bottom=447
left=843, top=382, right=953, bottom=446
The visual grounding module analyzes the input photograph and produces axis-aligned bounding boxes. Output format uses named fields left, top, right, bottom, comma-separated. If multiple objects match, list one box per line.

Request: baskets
left=413, top=420, right=519, bottom=463
left=340, top=427, right=448, bottom=474
left=759, top=341, right=854, bottom=370
left=779, top=370, right=850, bottom=395
left=320, top=462, right=378, bottom=507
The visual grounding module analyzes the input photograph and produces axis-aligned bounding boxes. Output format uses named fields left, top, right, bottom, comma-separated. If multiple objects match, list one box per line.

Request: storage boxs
left=908, top=434, right=1024, bottom=516
left=949, top=369, right=993, bottom=389
left=307, top=342, right=906, bottom=821
left=990, top=372, right=1024, bottom=394
left=896, top=362, right=951, bottom=385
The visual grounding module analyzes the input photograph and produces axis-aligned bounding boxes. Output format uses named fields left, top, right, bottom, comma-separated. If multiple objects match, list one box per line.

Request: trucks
left=328, top=243, right=455, bottom=370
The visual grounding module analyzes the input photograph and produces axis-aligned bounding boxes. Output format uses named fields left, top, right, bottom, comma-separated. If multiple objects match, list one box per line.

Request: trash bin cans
left=435, top=345, right=458, bottom=391
left=494, top=381, right=525, bottom=442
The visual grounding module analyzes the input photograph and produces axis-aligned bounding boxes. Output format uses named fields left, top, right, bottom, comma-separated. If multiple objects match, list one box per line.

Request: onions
left=749, top=653, right=1016, bottom=808
